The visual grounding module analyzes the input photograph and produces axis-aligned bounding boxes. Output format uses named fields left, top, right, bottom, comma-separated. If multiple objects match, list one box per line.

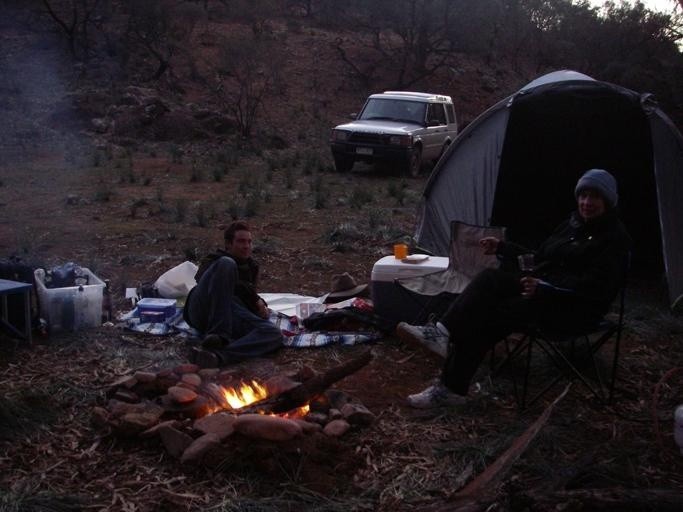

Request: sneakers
left=395, top=320, right=451, bottom=360
left=186, top=332, right=224, bottom=371
left=405, top=382, right=467, bottom=410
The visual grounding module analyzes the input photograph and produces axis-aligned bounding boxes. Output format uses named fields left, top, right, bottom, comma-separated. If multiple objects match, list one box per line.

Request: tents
left=407, top=67, right=682, bottom=313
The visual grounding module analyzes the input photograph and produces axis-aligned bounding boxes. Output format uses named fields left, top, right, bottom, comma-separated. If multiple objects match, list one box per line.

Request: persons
left=392, top=167, right=628, bottom=410
left=179, top=221, right=285, bottom=369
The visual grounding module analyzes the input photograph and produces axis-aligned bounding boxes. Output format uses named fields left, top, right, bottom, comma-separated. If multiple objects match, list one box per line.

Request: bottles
left=101, top=280, right=112, bottom=323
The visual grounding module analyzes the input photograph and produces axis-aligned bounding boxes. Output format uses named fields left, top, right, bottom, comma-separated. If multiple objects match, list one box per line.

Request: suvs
left=327, top=89, right=459, bottom=180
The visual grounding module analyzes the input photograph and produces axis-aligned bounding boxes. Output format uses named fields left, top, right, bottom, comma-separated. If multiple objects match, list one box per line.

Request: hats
left=573, top=168, right=618, bottom=209
left=323, top=272, right=369, bottom=299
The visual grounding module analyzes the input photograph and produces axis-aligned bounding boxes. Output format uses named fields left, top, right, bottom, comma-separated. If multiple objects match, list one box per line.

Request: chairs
left=495, top=247, right=628, bottom=409
left=394, top=224, right=518, bottom=349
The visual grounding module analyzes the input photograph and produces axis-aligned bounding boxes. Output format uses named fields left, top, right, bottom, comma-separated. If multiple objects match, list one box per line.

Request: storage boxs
left=34, top=261, right=106, bottom=338
left=136, top=297, right=177, bottom=324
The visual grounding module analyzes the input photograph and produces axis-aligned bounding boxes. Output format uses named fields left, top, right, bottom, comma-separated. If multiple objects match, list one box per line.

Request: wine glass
left=517, top=254, right=536, bottom=299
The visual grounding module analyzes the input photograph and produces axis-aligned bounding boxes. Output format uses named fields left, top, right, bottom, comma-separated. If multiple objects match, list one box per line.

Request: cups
left=395, top=245, right=408, bottom=259
